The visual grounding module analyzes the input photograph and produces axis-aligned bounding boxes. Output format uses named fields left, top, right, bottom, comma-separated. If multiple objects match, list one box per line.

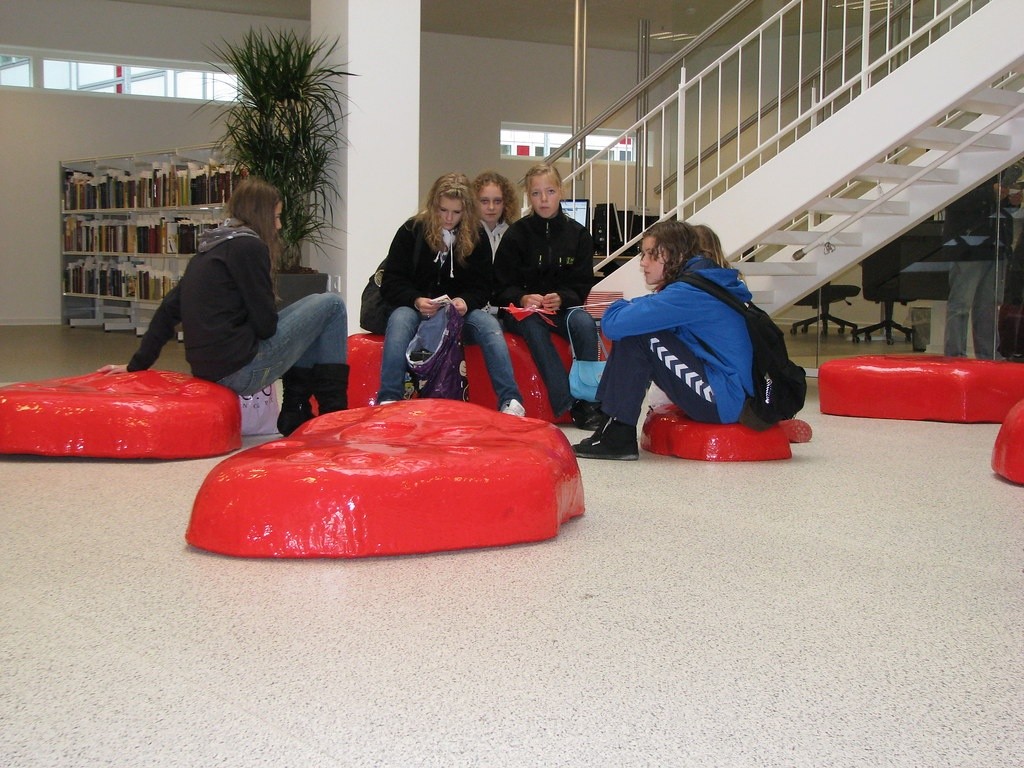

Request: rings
left=427, top=314, right=429, bottom=318
left=532, top=304, right=536, bottom=307
left=112, top=366, right=114, bottom=370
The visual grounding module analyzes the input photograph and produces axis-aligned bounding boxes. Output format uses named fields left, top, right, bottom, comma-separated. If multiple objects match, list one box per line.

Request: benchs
left=343, top=328, right=612, bottom=425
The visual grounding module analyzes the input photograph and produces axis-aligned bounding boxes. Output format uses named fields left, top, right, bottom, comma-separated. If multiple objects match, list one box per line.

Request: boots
left=277, top=366, right=318, bottom=437
left=311, top=363, right=350, bottom=415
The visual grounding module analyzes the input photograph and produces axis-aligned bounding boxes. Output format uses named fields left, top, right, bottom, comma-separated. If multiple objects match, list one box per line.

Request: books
left=64, top=169, right=246, bottom=299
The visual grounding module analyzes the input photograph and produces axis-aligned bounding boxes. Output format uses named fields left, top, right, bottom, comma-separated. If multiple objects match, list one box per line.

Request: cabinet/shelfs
left=59, top=131, right=299, bottom=338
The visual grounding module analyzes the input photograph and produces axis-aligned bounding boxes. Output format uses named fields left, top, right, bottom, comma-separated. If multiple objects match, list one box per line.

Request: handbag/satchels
left=568, top=309, right=610, bottom=402
left=238, top=381, right=281, bottom=435
left=360, top=257, right=390, bottom=334
left=405, top=303, right=468, bottom=400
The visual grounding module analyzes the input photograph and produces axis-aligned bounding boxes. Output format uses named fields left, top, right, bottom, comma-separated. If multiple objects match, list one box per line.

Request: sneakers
left=572, top=415, right=639, bottom=460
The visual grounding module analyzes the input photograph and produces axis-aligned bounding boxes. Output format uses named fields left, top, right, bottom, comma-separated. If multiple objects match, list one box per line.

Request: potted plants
left=194, top=29, right=360, bottom=311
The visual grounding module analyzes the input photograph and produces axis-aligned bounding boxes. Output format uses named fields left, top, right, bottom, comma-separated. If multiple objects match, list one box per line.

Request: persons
left=946, top=169, right=1024, bottom=360
left=96, top=178, right=350, bottom=436
left=570, top=220, right=754, bottom=461
left=375, top=164, right=607, bottom=431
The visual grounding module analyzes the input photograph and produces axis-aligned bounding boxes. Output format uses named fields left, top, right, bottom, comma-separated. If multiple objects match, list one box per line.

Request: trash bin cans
left=911, top=307, right=932, bottom=351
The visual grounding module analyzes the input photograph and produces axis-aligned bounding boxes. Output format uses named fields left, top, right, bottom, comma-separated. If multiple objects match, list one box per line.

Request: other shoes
left=570, top=400, right=609, bottom=430
left=500, top=399, right=526, bottom=417
left=380, top=400, right=397, bottom=405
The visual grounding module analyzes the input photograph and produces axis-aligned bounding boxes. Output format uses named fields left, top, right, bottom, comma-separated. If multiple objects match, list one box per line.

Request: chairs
left=789, top=277, right=860, bottom=337
left=852, top=264, right=916, bottom=346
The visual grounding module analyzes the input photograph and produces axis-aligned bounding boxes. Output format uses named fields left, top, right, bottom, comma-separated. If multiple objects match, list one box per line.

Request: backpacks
left=672, top=271, right=808, bottom=425
